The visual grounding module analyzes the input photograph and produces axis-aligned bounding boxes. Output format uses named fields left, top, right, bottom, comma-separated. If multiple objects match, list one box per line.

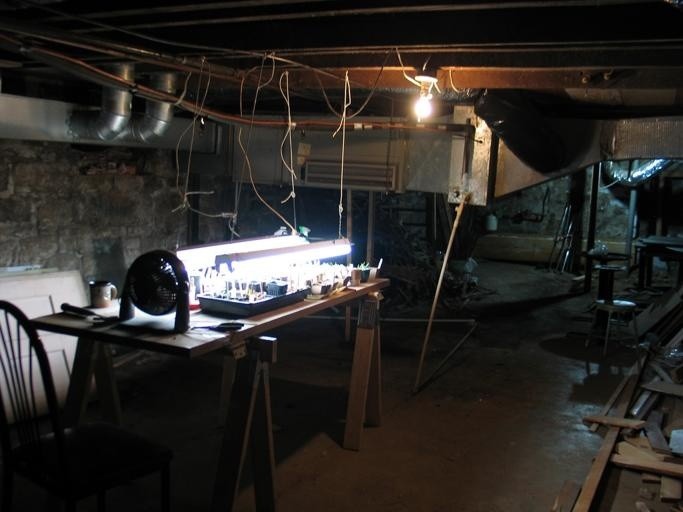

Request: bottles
left=288, top=263, right=299, bottom=293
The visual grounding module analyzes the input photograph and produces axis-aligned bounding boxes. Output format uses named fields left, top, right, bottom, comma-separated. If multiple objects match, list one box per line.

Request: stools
left=584, top=298, right=639, bottom=357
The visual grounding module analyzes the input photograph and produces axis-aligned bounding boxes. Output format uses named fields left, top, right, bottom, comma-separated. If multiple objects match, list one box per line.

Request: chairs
left=0, top=299, right=172, bottom=511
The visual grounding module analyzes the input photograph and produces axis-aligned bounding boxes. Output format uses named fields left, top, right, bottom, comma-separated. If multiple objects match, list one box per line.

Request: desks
left=639, top=235, right=683, bottom=292
left=29, top=276, right=390, bottom=512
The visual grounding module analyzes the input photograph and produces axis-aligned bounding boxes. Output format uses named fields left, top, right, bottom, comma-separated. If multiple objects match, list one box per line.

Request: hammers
left=61, top=303, right=119, bottom=327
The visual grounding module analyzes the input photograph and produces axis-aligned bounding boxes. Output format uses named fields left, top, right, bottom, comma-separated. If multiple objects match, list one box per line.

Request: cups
left=199, top=275, right=266, bottom=302
left=367, top=267, right=377, bottom=281
left=89, top=280, right=117, bottom=308
left=349, top=268, right=361, bottom=286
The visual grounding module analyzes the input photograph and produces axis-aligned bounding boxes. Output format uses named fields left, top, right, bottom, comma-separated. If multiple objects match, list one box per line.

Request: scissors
left=192, top=323, right=244, bottom=331
